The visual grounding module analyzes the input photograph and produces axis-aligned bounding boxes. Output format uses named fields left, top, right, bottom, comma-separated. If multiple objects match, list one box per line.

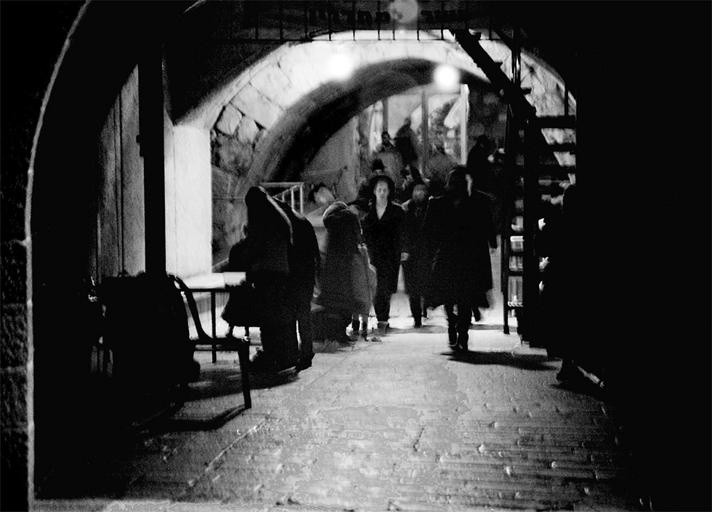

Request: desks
left=172, top=270, right=249, bottom=366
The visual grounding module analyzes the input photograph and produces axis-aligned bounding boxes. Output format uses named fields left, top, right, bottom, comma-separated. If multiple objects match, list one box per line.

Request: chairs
left=161, top=269, right=256, bottom=413
